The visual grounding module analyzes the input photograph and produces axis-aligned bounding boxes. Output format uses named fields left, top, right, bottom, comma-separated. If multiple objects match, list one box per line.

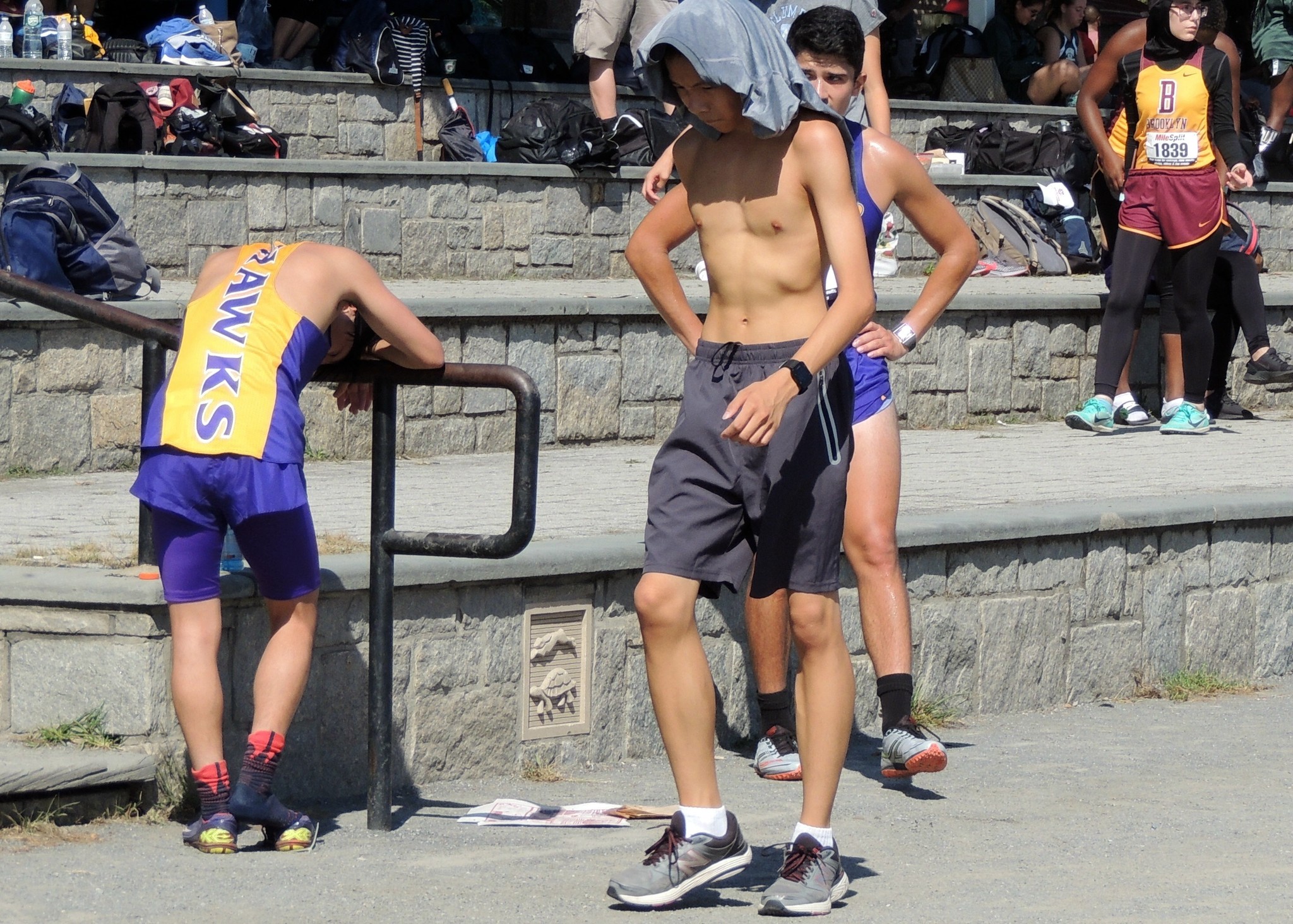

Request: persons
left=765, top=0, right=891, bottom=138
left=41, top=0, right=95, bottom=27
left=751, top=5, right=981, bottom=782
left=603, top=0, right=880, bottom=917
left=981, top=0, right=1101, bottom=107
left=271, top=0, right=322, bottom=70
left=1062, top=0, right=1293, bottom=436
left=560, top=0, right=679, bottom=169
left=130, top=241, right=445, bottom=855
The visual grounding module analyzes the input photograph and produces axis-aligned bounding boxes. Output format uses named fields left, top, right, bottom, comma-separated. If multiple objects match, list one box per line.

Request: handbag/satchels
left=190, top=14, right=246, bottom=68
left=196, top=73, right=260, bottom=126
left=602, top=107, right=683, bottom=166
left=940, top=23, right=1018, bottom=104
left=925, top=120, right=1095, bottom=175
left=330, top=16, right=431, bottom=98
left=495, top=97, right=621, bottom=174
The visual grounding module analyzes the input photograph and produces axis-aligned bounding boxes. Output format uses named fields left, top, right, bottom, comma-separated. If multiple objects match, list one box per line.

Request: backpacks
left=0, top=95, right=51, bottom=150
left=969, top=182, right=1100, bottom=277
left=137, top=78, right=199, bottom=129
left=84, top=80, right=157, bottom=155
left=0, top=161, right=160, bottom=301
left=51, top=82, right=89, bottom=153
left=157, top=107, right=288, bottom=158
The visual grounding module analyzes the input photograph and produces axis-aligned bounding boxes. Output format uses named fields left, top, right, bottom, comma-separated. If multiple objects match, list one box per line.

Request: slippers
left=1113, top=401, right=1157, bottom=426
left=1160, top=413, right=1217, bottom=426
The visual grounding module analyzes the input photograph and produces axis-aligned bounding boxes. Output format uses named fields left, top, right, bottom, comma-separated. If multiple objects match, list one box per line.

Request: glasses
left=1171, top=5, right=1208, bottom=18
left=1027, top=7, right=1039, bottom=18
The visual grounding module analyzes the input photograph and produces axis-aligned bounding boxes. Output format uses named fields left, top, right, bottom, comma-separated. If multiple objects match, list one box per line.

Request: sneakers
left=1242, top=348, right=1293, bottom=385
left=1159, top=401, right=1210, bottom=435
left=607, top=810, right=752, bottom=909
left=1065, top=398, right=1113, bottom=433
left=261, top=815, right=315, bottom=853
left=161, top=41, right=182, bottom=65
left=757, top=832, right=849, bottom=917
left=970, top=260, right=990, bottom=276
left=180, top=40, right=234, bottom=66
left=1204, top=385, right=1254, bottom=419
left=181, top=812, right=239, bottom=855
left=977, top=248, right=1029, bottom=277
left=754, top=724, right=802, bottom=780
left=880, top=716, right=947, bottom=779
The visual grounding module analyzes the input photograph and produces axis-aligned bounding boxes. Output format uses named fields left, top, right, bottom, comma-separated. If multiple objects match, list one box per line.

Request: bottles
left=435, top=32, right=454, bottom=55
left=22, top=0, right=43, bottom=59
left=221, top=523, right=244, bottom=571
left=198, top=5, right=214, bottom=24
left=8, top=79, right=35, bottom=110
left=57, top=16, right=72, bottom=60
left=1055, top=120, right=1071, bottom=132
left=0, top=17, right=13, bottom=58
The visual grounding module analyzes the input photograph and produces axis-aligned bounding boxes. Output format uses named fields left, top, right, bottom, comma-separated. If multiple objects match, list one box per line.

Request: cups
left=443, top=59, right=457, bottom=75
left=695, top=260, right=708, bottom=281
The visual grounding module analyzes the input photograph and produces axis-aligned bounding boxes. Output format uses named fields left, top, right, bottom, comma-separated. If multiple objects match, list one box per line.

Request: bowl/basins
left=931, top=164, right=963, bottom=175
left=914, top=153, right=934, bottom=172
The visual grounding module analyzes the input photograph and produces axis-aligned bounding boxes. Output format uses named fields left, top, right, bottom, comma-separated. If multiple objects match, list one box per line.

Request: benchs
left=0, top=58, right=1293, bottom=321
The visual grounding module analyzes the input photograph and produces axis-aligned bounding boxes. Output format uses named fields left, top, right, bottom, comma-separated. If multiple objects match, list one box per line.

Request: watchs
left=891, top=322, right=916, bottom=352
left=780, top=359, right=813, bottom=395
